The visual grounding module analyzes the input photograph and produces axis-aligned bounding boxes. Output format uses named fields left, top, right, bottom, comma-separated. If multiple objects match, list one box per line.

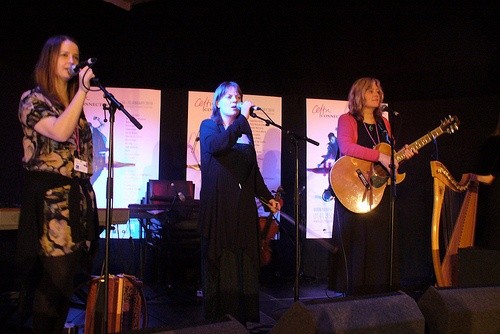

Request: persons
left=197, top=82, right=281, bottom=327
left=18, top=35, right=100, bottom=334
left=329, top=78, right=418, bottom=294
left=328, top=133, right=339, bottom=159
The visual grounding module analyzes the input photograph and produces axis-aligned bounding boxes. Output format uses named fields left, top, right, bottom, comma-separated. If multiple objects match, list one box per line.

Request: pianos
left=0, top=207, right=130, bottom=231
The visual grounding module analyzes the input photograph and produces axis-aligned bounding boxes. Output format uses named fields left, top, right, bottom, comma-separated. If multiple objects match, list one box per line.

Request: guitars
left=330, top=115, right=459, bottom=214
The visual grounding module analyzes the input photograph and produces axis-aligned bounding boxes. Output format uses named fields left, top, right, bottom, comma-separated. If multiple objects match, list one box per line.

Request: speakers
left=266, top=249, right=500, bottom=334
left=155, top=314, right=249, bottom=334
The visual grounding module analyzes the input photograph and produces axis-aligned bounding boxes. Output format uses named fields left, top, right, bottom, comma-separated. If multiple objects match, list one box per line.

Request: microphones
left=68, top=57, right=98, bottom=76
left=237, top=102, right=262, bottom=110
left=382, top=104, right=399, bottom=116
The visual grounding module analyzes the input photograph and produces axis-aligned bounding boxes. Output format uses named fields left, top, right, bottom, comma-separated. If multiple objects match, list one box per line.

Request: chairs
left=146, top=180, right=201, bottom=293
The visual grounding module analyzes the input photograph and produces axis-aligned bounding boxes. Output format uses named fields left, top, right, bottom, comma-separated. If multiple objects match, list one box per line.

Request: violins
left=258, top=186, right=284, bottom=267
